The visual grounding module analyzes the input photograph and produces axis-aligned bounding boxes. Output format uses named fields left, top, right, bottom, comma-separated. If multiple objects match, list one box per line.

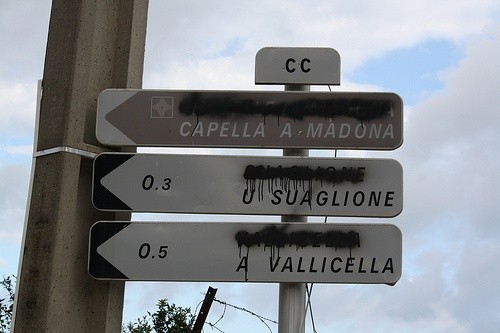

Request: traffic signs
left=87, top=47, right=404, bottom=286
